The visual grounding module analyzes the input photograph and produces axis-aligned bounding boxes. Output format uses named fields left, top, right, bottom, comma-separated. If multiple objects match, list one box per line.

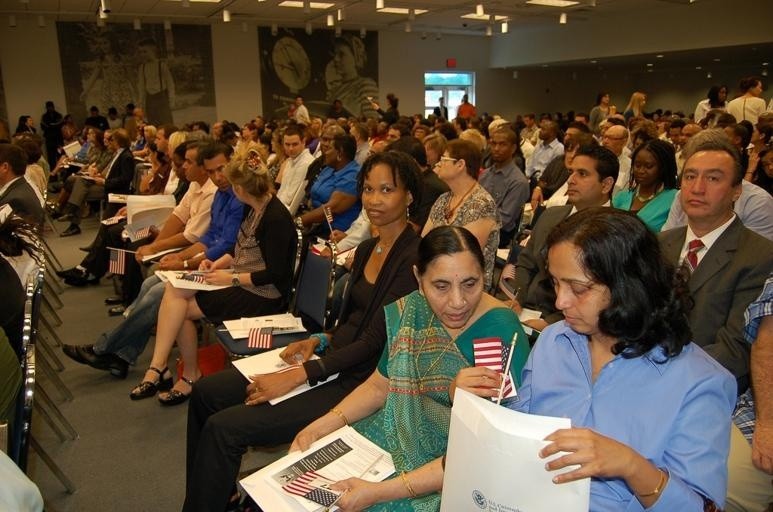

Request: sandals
left=130, top=366, right=174, bottom=400
left=158, top=372, right=202, bottom=406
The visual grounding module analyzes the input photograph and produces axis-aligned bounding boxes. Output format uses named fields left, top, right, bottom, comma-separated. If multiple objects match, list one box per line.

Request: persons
left=294, top=35, right=381, bottom=119
left=78, top=33, right=138, bottom=121
left=135, top=40, right=178, bottom=130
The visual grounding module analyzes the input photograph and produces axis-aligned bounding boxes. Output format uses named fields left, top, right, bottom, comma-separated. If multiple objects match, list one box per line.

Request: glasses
left=439, top=155, right=454, bottom=162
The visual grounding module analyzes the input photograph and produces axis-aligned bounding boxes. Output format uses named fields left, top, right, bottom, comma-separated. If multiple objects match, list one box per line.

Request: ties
left=683, top=239, right=703, bottom=281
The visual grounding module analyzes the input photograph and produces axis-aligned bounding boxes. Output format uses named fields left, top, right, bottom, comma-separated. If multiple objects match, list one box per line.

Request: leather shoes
left=60, top=343, right=105, bottom=370
left=64, top=271, right=100, bottom=287
left=46, top=171, right=90, bottom=236
left=108, top=305, right=125, bottom=315
left=79, top=243, right=94, bottom=251
left=105, top=296, right=122, bottom=304
left=88, top=351, right=130, bottom=378
left=55, top=266, right=89, bottom=282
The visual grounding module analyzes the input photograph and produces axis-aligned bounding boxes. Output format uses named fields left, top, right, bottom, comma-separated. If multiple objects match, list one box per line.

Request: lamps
left=279, top=1, right=334, bottom=10
left=327, top=14, right=334, bottom=27
left=476, top=4, right=483, bottom=15
left=528, top=1, right=578, bottom=7
left=376, top=0, right=384, bottom=8
left=100, top=7, right=107, bottom=18
left=378, top=7, right=428, bottom=15
left=103, top=1, right=109, bottom=13
left=338, top=9, right=344, bottom=21
left=502, top=22, right=507, bottom=33
left=461, top=13, right=508, bottom=21
left=224, top=11, right=230, bottom=22
left=560, top=13, right=567, bottom=24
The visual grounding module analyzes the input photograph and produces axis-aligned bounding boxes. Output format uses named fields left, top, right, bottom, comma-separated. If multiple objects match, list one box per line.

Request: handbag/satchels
left=175, top=343, right=225, bottom=381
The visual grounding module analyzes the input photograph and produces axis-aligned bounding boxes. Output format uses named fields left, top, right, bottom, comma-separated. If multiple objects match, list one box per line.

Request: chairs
left=212, top=235, right=337, bottom=373
left=13, top=168, right=77, bottom=496
